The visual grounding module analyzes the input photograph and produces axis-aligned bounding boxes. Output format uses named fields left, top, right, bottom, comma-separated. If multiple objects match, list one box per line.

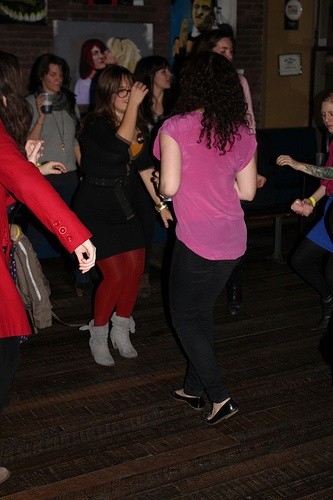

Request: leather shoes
left=308, top=296, right=333, bottom=335
left=225, top=276, right=241, bottom=316
left=171, top=387, right=204, bottom=409
left=204, top=398, right=240, bottom=425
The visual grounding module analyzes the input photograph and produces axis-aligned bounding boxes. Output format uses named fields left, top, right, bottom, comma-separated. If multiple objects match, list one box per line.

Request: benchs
left=247, top=126, right=319, bottom=265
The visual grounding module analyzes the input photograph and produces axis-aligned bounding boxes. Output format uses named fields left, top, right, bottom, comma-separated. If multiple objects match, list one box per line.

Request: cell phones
left=291, top=199, right=313, bottom=218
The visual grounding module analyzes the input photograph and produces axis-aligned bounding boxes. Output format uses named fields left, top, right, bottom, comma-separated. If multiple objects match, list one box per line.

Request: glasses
left=111, top=90, right=131, bottom=98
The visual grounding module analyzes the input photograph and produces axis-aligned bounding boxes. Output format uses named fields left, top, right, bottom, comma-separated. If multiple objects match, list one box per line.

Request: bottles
left=227, top=276, right=241, bottom=315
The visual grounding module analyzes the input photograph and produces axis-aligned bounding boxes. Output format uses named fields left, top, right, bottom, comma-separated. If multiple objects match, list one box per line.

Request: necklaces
left=49, top=110, right=65, bottom=154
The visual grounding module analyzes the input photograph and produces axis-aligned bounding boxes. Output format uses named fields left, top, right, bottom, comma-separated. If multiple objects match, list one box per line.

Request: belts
left=82, top=175, right=132, bottom=188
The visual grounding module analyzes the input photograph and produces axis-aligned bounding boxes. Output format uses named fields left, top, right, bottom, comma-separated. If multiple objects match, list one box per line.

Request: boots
left=89, top=318, right=115, bottom=366
left=112, top=314, right=136, bottom=358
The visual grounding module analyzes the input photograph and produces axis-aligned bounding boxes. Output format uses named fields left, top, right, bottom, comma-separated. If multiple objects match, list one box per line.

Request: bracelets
left=155, top=201, right=168, bottom=212
left=308, top=195, right=316, bottom=207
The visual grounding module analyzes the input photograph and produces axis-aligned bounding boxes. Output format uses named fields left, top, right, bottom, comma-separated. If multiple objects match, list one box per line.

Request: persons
left=0, top=0, right=267, bottom=427
left=275, top=97, right=332, bottom=365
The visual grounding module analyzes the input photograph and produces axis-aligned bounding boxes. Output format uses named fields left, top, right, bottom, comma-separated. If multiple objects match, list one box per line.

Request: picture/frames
left=277, top=53, right=303, bottom=77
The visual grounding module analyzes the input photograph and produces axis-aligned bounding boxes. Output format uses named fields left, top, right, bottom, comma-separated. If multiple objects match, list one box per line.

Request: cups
left=39, top=93, right=55, bottom=114
